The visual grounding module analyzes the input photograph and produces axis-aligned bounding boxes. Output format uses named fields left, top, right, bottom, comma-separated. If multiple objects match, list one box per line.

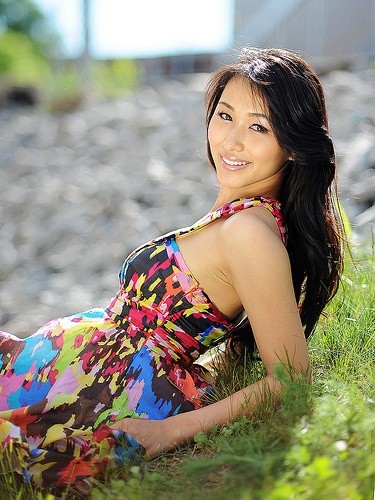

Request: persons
left=1, top=45, right=344, bottom=500
